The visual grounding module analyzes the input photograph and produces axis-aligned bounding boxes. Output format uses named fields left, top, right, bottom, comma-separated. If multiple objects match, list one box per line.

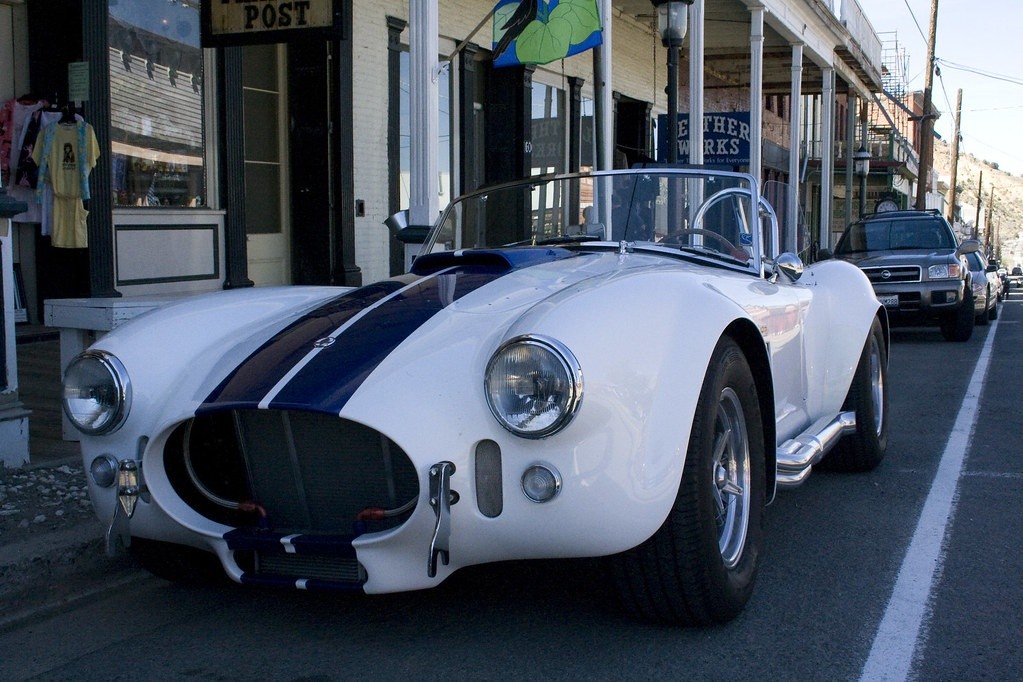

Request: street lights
left=646, top=0, right=695, bottom=246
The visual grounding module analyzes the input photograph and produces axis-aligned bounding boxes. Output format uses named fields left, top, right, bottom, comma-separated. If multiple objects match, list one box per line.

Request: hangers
left=15, top=87, right=77, bottom=124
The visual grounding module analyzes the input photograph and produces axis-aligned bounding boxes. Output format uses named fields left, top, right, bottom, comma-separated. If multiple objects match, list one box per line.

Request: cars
left=960, top=249, right=1023, bottom=324
left=153, top=176, right=186, bottom=205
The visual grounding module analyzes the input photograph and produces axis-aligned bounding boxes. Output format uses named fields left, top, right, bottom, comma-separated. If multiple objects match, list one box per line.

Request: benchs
left=43, top=297, right=201, bottom=441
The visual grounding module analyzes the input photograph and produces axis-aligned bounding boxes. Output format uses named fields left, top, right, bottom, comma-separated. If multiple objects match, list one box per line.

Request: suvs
left=832, top=209, right=976, bottom=342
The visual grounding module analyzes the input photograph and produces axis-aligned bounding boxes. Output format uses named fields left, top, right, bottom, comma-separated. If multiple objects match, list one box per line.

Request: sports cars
left=59, top=164, right=897, bottom=627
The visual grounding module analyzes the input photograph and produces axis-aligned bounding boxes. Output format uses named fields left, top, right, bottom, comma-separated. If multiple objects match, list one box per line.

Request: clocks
left=875, top=200, right=899, bottom=213
